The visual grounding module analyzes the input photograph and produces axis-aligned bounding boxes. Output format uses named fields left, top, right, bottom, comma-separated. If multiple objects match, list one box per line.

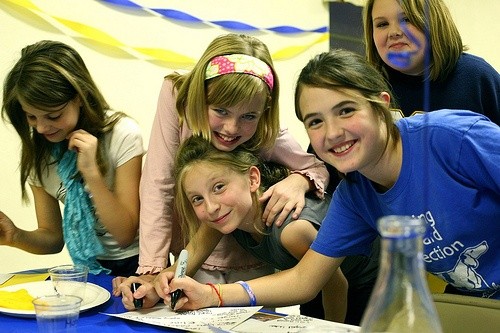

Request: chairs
left=371, top=293, right=500, bottom=333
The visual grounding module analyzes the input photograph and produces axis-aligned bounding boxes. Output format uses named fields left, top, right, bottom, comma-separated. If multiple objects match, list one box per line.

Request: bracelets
left=206, top=280, right=224, bottom=306
left=235, top=280, right=258, bottom=306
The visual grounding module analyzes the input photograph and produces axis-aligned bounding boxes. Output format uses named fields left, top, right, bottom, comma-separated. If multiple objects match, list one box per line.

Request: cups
left=32, top=295, right=84, bottom=333
left=48, top=264, right=90, bottom=303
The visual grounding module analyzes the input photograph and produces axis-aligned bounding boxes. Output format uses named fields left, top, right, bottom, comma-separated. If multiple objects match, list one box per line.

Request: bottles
left=359, top=214, right=442, bottom=333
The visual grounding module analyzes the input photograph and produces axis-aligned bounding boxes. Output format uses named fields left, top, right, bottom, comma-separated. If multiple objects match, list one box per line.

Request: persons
left=112, top=34, right=330, bottom=298
left=119, top=136, right=410, bottom=333
left=364, top=1, right=500, bottom=127
left=159, top=50, right=500, bottom=310
left=0, top=41, right=147, bottom=276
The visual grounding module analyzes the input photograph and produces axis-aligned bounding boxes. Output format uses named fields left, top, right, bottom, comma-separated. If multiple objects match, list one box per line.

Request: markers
left=169, top=250, right=188, bottom=311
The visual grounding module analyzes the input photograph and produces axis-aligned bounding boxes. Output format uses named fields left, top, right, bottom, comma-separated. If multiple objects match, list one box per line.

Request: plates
left=0, top=280, right=111, bottom=318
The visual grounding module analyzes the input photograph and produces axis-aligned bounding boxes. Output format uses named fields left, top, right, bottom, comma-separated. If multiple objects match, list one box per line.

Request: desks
left=0, top=267, right=288, bottom=333
